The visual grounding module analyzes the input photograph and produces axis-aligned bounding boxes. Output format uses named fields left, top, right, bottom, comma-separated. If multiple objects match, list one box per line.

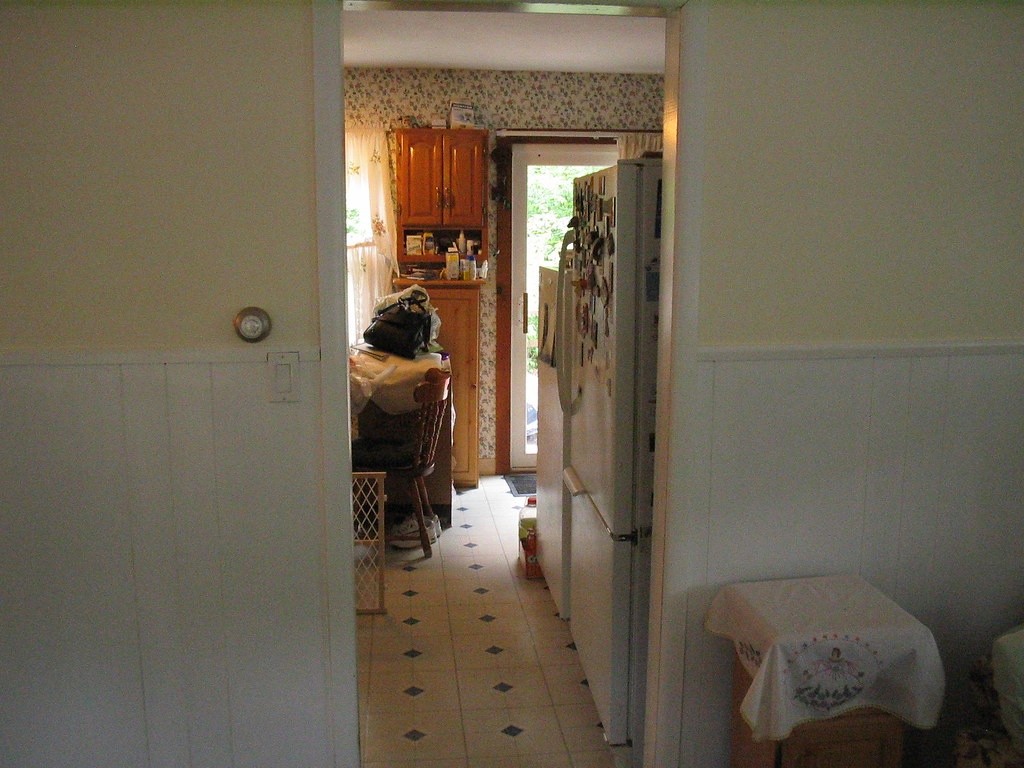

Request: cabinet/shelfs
left=392, top=279, right=486, bottom=488
left=704, top=574, right=946, bottom=768
left=396, top=128, right=489, bottom=262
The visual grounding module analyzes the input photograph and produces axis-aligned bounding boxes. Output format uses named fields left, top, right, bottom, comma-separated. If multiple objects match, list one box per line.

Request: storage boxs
left=432, top=119, right=447, bottom=129
left=449, top=98, right=474, bottom=129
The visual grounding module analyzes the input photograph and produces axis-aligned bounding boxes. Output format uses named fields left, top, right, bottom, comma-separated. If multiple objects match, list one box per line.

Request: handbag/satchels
left=364, top=290, right=431, bottom=360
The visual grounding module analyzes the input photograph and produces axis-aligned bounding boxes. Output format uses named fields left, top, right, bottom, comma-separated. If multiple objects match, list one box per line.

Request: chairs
left=351, top=367, right=451, bottom=560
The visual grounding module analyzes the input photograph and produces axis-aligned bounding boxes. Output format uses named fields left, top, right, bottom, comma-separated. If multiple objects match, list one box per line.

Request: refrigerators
left=554, top=156, right=661, bottom=748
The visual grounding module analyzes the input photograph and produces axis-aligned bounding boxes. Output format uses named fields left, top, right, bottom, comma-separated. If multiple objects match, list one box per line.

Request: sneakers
left=388, top=513, right=442, bottom=548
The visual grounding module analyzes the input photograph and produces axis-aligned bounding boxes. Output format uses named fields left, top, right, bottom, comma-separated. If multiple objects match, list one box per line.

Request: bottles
left=457, top=231, right=488, bottom=280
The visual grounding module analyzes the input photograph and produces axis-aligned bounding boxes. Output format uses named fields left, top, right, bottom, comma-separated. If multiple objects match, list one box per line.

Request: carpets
left=504, top=472, right=537, bottom=497
